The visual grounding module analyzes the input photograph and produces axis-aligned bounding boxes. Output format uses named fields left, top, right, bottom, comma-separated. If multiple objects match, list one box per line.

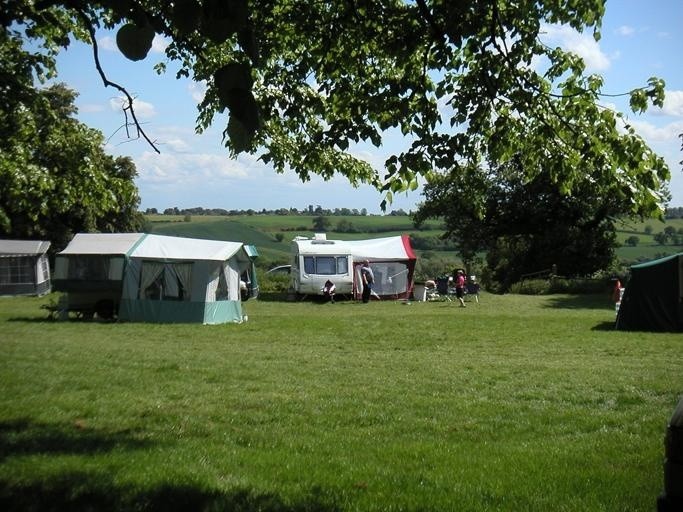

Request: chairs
left=465, top=283, right=479, bottom=302
left=437, top=278, right=452, bottom=302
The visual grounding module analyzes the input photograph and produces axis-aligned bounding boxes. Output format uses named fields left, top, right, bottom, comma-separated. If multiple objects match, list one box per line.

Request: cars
left=263, top=264, right=291, bottom=277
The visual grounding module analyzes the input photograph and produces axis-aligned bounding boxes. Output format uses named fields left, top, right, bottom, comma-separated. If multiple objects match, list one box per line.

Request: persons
left=359, top=259, right=375, bottom=303
left=452, top=270, right=466, bottom=307
left=466, top=281, right=480, bottom=294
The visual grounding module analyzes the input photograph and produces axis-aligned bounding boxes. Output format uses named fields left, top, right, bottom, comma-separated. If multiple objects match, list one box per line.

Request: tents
left=614, top=252, right=682, bottom=333
left=0, top=239, right=49, bottom=296
left=49, top=232, right=260, bottom=326
left=291, top=232, right=414, bottom=300
